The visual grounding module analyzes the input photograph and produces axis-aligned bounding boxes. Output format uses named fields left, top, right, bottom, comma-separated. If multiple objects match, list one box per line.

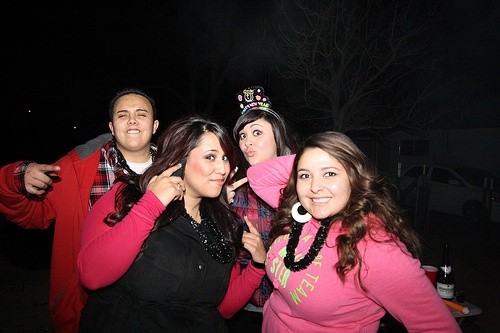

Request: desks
left=446, top=296, right=482, bottom=327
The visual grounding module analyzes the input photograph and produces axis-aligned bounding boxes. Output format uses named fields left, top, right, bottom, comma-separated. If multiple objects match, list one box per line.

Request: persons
left=218, top=106, right=300, bottom=333
left=78, top=116, right=267, bottom=333
left=247, top=131, right=462, bottom=333
left=0, top=89, right=159, bottom=333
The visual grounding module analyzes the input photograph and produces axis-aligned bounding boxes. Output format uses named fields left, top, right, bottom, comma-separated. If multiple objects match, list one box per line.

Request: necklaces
left=284, top=206, right=334, bottom=272
left=112, top=137, right=156, bottom=175
left=183, top=207, right=232, bottom=264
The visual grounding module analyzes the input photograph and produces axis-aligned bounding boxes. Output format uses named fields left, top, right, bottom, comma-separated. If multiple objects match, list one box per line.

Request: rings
left=176, top=183, right=180, bottom=189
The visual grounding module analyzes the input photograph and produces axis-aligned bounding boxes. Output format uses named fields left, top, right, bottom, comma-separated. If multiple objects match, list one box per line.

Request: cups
left=422, top=265, right=439, bottom=288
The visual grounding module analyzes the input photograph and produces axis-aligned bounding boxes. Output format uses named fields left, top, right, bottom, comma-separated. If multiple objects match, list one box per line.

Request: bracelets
left=251, top=259, right=266, bottom=269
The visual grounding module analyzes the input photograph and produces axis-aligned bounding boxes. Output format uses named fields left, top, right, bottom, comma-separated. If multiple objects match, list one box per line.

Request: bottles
left=436, top=245, right=456, bottom=301
